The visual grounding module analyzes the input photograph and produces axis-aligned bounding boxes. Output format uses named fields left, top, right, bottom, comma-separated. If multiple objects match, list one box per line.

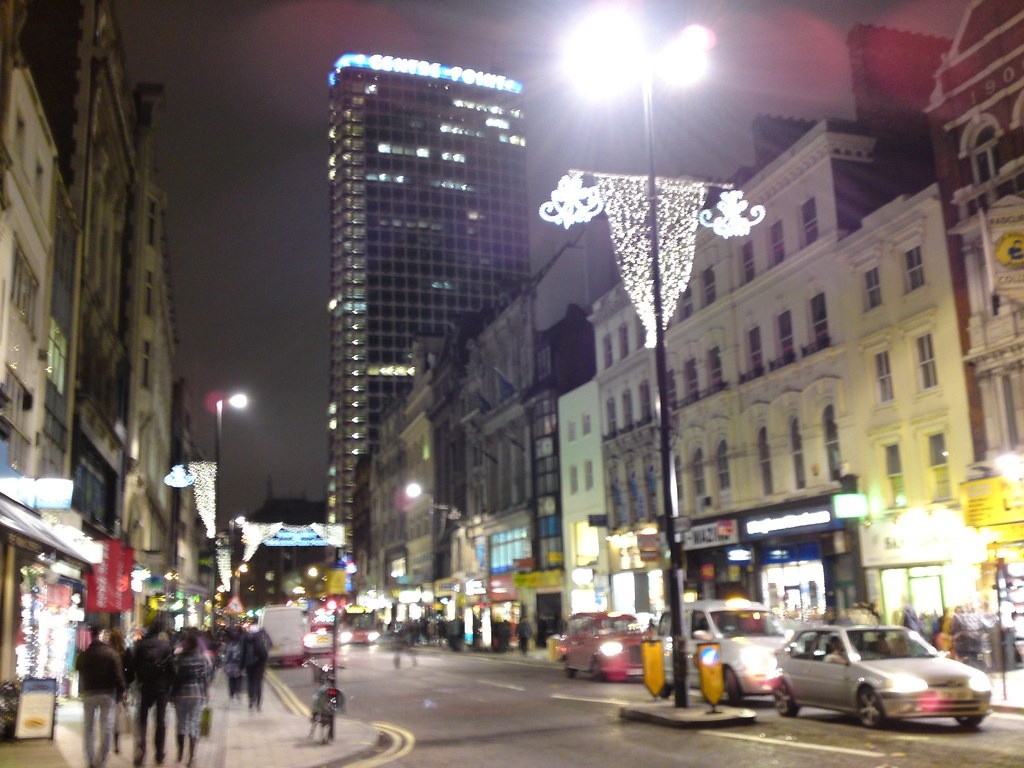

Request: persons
left=377, top=615, right=566, bottom=655
left=76, top=615, right=272, bottom=768
left=825, top=602, right=987, bottom=674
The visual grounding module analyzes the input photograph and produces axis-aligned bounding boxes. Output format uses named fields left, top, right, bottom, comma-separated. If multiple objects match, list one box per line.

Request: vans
left=259, top=606, right=304, bottom=665
left=653, top=599, right=793, bottom=705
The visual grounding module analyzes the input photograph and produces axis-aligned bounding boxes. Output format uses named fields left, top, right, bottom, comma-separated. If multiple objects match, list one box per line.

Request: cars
left=769, top=625, right=993, bottom=729
left=558, top=612, right=655, bottom=684
left=305, top=622, right=338, bottom=656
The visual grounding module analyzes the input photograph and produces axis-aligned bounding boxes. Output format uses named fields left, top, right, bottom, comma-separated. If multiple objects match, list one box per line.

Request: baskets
left=313, top=666, right=334, bottom=684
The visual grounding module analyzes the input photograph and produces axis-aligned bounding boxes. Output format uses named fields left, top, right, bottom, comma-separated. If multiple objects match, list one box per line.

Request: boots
left=186, top=734, right=197, bottom=767
left=113, top=730, right=120, bottom=753
left=176, top=733, right=185, bottom=761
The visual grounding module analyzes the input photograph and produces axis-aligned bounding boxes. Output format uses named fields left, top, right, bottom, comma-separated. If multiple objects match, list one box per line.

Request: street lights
left=539, top=4, right=768, bottom=709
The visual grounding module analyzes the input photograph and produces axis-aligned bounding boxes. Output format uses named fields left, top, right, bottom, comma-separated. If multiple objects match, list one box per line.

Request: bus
left=340, top=606, right=378, bottom=646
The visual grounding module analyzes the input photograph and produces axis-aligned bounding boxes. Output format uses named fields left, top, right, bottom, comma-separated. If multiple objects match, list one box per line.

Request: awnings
left=0, top=492, right=94, bottom=574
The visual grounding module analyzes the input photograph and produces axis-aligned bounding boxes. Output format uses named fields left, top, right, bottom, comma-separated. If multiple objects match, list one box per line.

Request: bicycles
left=301, top=658, right=346, bottom=744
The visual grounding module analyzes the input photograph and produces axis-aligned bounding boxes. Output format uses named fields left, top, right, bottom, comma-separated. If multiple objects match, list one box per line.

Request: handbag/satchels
left=200, top=700, right=213, bottom=736
left=122, top=695, right=133, bottom=736
